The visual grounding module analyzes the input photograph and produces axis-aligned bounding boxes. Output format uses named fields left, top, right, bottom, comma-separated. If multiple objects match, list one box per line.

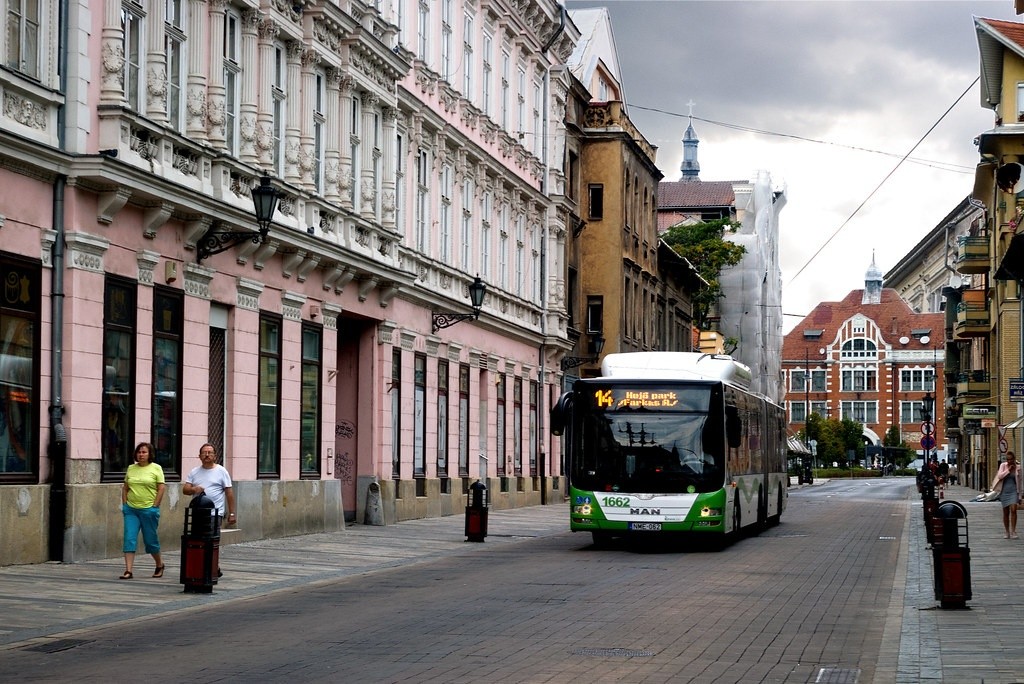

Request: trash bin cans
left=463, top=479, right=488, bottom=542
left=916, top=471, right=941, bottom=550
left=930, top=500, right=972, bottom=610
left=179, top=491, right=223, bottom=593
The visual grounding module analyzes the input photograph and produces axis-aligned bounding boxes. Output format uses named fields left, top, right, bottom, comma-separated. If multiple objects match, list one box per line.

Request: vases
left=1014, top=215, right=1024, bottom=234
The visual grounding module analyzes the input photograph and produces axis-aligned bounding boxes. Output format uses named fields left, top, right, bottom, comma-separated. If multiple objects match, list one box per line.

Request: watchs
left=229, top=513, right=235, bottom=517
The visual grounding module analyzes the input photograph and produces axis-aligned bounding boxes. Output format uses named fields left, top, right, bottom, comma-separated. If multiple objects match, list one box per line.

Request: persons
left=934, top=459, right=957, bottom=487
left=997, top=452, right=1022, bottom=540
left=119, top=443, right=165, bottom=579
left=182, top=444, right=236, bottom=582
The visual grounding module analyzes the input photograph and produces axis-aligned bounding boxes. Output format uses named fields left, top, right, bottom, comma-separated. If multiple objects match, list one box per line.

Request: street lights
left=922, top=388, right=934, bottom=468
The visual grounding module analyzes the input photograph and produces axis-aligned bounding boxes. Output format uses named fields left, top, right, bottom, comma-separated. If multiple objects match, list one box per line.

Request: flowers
left=1008, top=206, right=1024, bottom=230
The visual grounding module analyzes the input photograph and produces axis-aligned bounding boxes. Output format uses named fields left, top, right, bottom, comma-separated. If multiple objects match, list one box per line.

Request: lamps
left=431, top=272, right=486, bottom=335
left=561, top=335, right=606, bottom=383
left=197, top=169, right=279, bottom=263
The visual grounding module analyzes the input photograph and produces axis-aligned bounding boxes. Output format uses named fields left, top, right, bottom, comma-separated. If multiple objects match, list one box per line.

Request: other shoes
left=217, top=568, right=223, bottom=577
left=1003, top=533, right=1010, bottom=538
left=1012, top=532, right=1019, bottom=539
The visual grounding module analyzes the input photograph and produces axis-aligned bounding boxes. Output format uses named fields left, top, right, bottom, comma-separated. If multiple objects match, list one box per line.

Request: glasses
left=201, top=452, right=214, bottom=455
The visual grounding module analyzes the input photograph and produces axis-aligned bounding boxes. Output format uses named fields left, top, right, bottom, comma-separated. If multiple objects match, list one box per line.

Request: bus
left=550, top=352, right=790, bottom=547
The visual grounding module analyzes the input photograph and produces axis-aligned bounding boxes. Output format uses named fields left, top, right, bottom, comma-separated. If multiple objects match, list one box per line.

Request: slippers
left=153, top=563, right=164, bottom=577
left=120, top=571, right=133, bottom=579
